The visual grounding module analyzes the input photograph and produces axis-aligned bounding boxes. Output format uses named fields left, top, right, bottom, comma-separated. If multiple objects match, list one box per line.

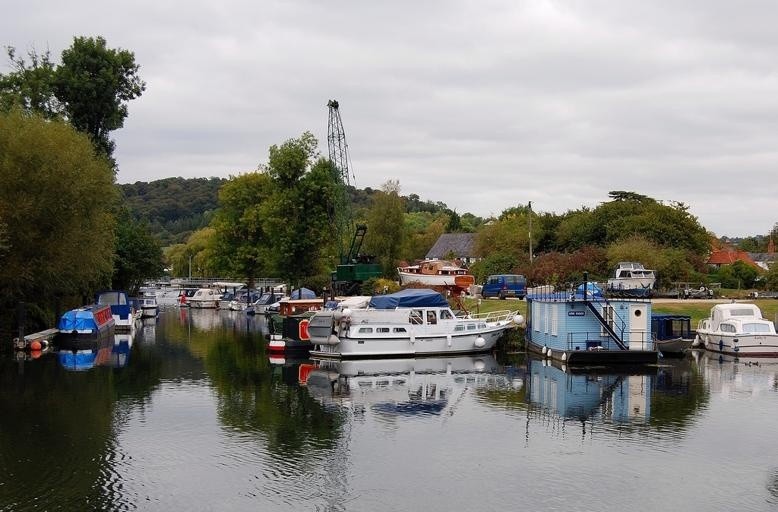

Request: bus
left=659, top=282, right=721, bottom=299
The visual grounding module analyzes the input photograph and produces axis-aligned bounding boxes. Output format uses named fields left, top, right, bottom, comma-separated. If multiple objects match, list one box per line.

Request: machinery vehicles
left=680, top=287, right=713, bottom=298
left=330, top=225, right=384, bottom=295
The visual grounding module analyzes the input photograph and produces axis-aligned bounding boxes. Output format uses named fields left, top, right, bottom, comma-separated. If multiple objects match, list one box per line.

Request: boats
left=158, top=288, right=223, bottom=308
left=397, top=257, right=474, bottom=292
left=265, top=311, right=317, bottom=352
left=309, top=289, right=519, bottom=357
left=59, top=334, right=133, bottom=373
left=52, top=305, right=115, bottom=344
left=651, top=314, right=694, bottom=353
left=228, top=289, right=321, bottom=316
left=95, top=290, right=159, bottom=331
left=697, top=304, right=778, bottom=358
left=607, top=261, right=656, bottom=296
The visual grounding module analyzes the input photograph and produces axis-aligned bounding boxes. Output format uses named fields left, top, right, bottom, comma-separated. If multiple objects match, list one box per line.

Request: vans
left=482, top=274, right=527, bottom=300
